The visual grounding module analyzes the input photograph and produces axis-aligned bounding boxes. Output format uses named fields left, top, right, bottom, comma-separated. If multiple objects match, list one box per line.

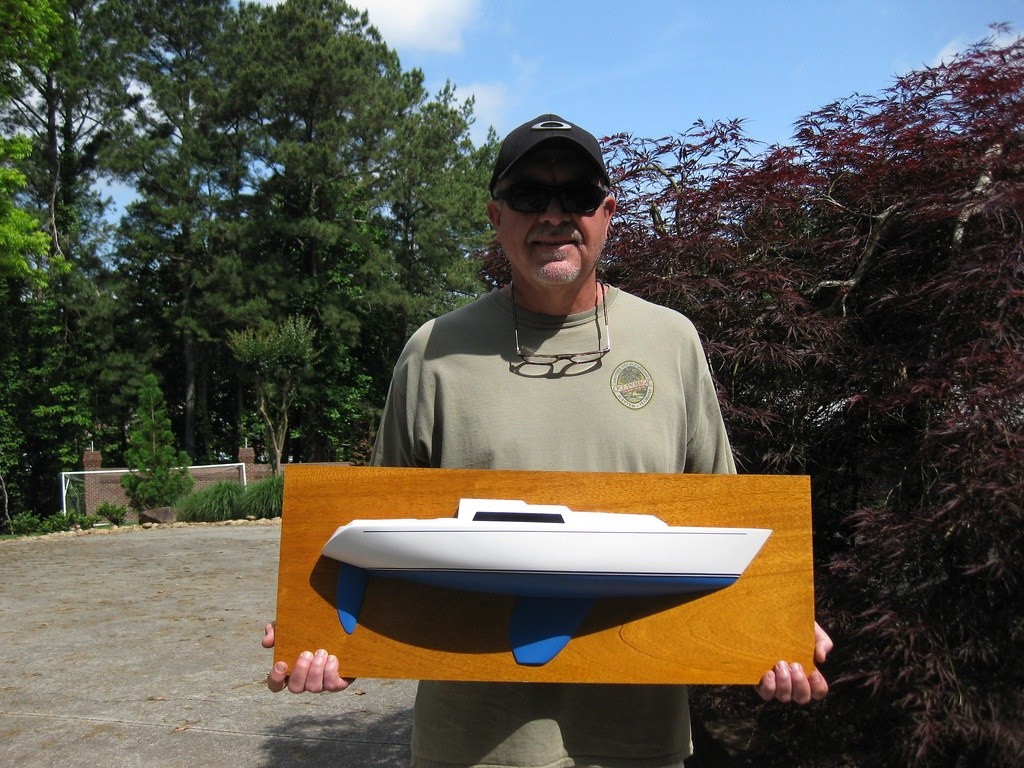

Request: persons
left=262, top=114, right=834, bottom=768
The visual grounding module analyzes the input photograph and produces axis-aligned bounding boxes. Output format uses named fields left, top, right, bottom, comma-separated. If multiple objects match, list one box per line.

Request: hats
left=489, top=114, right=610, bottom=200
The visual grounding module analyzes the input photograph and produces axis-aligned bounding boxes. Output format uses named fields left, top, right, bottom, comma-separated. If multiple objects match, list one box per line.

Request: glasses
left=494, top=181, right=609, bottom=217
left=510, top=281, right=610, bottom=365
